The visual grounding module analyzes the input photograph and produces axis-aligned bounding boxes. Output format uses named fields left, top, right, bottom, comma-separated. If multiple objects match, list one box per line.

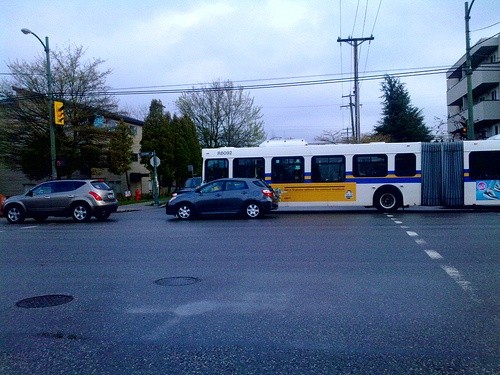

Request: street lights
left=21, top=27, right=58, bottom=179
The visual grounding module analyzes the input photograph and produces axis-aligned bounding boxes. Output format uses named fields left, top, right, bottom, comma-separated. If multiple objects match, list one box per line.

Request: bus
left=200, top=134, right=500, bottom=214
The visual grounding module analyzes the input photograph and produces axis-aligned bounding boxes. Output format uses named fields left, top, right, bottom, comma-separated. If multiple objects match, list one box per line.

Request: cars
left=0, top=193, right=6, bottom=217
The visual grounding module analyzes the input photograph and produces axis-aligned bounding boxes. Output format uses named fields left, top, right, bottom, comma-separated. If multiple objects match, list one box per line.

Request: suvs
left=165, top=177, right=281, bottom=220
left=2, top=179, right=119, bottom=224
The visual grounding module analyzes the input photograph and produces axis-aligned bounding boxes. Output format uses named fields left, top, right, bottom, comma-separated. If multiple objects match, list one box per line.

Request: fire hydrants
left=135, top=188, right=142, bottom=202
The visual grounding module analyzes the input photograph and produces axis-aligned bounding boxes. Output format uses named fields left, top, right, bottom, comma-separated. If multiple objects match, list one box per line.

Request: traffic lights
left=463, top=127, right=467, bottom=137
left=53, top=100, right=65, bottom=126
